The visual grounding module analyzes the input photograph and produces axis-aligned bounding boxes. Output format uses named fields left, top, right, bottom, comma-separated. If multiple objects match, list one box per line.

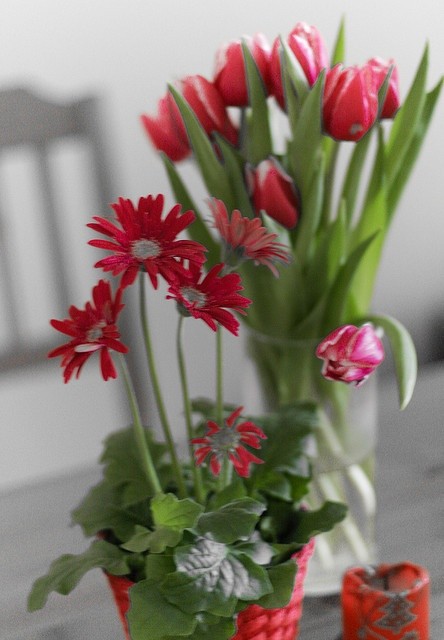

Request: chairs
left=0, top=79, right=150, bottom=450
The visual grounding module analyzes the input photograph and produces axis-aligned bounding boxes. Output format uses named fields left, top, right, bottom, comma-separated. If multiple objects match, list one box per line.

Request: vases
left=96, top=534, right=318, bottom=640
left=227, top=320, right=381, bottom=599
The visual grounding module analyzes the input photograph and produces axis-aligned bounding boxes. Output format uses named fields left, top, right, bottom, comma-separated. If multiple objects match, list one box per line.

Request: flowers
left=137, top=12, right=443, bottom=453
left=25, top=191, right=350, bottom=640
